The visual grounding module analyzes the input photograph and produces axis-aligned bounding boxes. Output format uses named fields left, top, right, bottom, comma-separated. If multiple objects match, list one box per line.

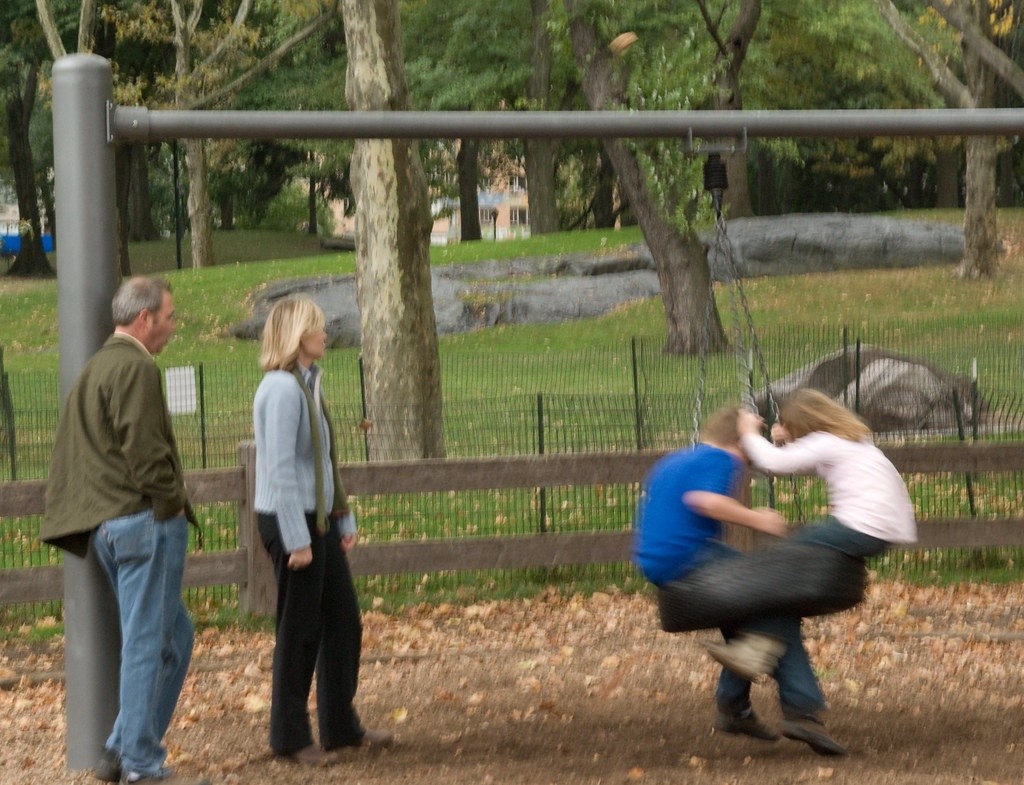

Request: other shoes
left=714, top=707, right=781, bottom=742
left=361, top=728, right=395, bottom=747
left=782, top=706, right=848, bottom=758
left=95, top=747, right=122, bottom=780
left=120, top=774, right=208, bottom=785
left=292, top=746, right=338, bottom=766
left=706, top=632, right=789, bottom=684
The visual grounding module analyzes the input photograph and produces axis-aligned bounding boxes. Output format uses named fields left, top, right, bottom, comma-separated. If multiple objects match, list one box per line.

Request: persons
left=254, top=293, right=394, bottom=763
left=633, top=409, right=847, bottom=757
left=657, top=386, right=920, bottom=682
left=37, top=274, right=197, bottom=783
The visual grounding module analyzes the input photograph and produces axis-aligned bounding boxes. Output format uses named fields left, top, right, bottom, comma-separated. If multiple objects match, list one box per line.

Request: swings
left=654, top=154, right=869, bottom=635
